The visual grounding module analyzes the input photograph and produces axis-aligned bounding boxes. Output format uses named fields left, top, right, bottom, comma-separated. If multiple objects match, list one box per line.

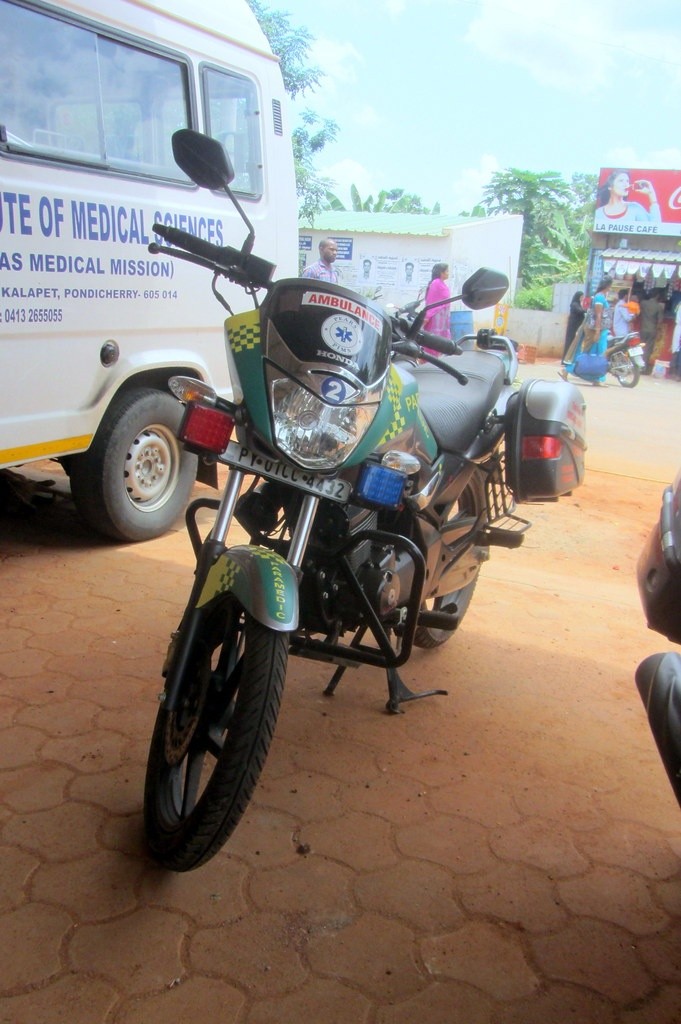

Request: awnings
left=599, top=248, right=681, bottom=279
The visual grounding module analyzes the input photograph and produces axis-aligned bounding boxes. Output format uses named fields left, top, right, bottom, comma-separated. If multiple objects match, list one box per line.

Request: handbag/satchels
left=586, top=297, right=614, bottom=330
left=574, top=342, right=608, bottom=379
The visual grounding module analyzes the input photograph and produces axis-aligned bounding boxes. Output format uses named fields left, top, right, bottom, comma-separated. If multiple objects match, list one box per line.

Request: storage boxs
left=636, top=479, right=681, bottom=643
left=517, top=345, right=536, bottom=364
left=504, top=379, right=585, bottom=503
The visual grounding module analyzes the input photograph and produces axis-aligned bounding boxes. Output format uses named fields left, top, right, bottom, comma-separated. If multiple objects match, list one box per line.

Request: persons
left=557, top=275, right=612, bottom=386
left=638, top=288, right=664, bottom=374
left=618, top=295, right=640, bottom=316
left=418, top=263, right=450, bottom=365
left=405, top=263, right=414, bottom=282
left=613, top=290, right=634, bottom=341
left=363, top=259, right=372, bottom=279
left=672, top=291, right=681, bottom=381
left=561, top=291, right=585, bottom=365
left=300, top=239, right=344, bottom=285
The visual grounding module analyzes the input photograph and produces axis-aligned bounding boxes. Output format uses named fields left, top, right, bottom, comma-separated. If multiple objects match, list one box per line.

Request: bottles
left=625, top=182, right=644, bottom=191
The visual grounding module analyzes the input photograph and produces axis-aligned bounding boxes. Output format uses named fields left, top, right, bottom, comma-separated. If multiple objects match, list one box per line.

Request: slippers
left=558, top=371, right=569, bottom=382
left=593, top=383, right=608, bottom=387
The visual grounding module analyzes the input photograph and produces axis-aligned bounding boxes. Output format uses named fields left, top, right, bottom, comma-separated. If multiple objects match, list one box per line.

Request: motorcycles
left=136, top=128, right=583, bottom=881
left=631, top=470, right=681, bottom=813
left=561, top=295, right=645, bottom=389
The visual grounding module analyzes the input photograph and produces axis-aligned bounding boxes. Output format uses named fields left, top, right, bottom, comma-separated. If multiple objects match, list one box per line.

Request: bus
left=1, top=1, right=299, bottom=546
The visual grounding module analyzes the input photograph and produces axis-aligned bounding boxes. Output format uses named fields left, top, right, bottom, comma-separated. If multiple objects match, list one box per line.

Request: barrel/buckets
left=450, top=310, right=474, bottom=346
